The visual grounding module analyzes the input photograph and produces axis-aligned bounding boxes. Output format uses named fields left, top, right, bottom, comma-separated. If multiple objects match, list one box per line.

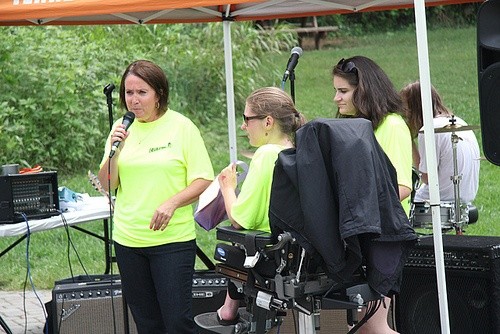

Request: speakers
left=477, top=1, right=500, bottom=166
left=394, top=234, right=500, bottom=334
left=189, top=270, right=246, bottom=334
left=53, top=275, right=139, bottom=334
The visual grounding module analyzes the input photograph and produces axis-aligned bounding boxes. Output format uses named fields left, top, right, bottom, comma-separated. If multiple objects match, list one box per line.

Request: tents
left=0, top=1, right=483, bottom=334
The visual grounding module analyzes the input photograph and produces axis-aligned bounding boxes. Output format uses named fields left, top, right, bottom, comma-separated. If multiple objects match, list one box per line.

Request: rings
left=222, top=175, right=226, bottom=178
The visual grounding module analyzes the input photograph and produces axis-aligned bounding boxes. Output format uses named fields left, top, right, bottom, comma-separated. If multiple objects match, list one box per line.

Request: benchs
left=256, top=25, right=338, bottom=51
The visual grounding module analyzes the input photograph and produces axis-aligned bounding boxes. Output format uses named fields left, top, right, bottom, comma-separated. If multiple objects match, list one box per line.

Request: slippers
left=216, top=304, right=243, bottom=325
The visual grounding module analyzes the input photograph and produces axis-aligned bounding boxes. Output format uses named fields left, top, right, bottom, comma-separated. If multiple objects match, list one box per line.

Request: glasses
left=242, top=113, right=267, bottom=127
left=335, top=56, right=359, bottom=80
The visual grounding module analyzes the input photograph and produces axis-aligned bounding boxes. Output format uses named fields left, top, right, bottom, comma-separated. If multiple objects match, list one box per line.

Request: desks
left=0, top=197, right=117, bottom=334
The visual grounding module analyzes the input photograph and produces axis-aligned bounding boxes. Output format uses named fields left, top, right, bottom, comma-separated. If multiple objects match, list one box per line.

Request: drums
left=410, top=199, right=453, bottom=230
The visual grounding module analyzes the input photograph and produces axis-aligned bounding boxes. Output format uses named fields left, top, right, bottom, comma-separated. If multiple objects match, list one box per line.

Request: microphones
left=109, top=112, right=135, bottom=158
left=283, top=47, right=303, bottom=81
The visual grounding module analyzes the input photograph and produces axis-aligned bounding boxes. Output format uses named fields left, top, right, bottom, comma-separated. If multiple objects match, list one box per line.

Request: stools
left=467, top=205, right=478, bottom=226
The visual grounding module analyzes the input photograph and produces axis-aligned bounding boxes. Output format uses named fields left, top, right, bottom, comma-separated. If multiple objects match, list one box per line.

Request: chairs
left=194, top=118, right=401, bottom=334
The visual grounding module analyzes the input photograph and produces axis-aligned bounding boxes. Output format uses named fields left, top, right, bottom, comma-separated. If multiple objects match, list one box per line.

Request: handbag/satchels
left=193, top=160, right=249, bottom=231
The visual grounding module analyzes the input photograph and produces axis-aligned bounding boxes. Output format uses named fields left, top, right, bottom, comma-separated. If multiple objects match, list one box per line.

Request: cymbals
left=418, top=124, right=477, bottom=134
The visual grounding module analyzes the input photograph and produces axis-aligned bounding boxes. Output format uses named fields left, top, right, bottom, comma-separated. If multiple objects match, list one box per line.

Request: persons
left=399, top=81, right=480, bottom=229
left=216, top=87, right=306, bottom=326
left=97, top=60, right=215, bottom=334
left=333, top=55, right=413, bottom=334
